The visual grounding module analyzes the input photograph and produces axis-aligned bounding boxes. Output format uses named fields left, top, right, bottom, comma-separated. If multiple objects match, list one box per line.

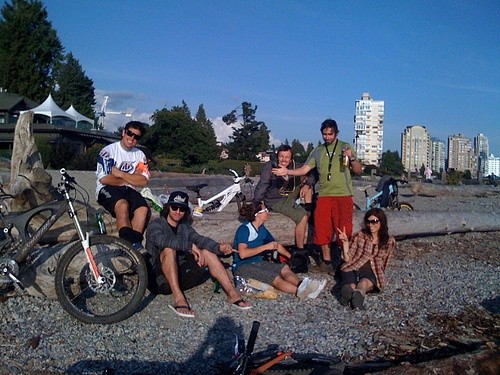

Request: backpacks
left=271, top=246, right=310, bottom=275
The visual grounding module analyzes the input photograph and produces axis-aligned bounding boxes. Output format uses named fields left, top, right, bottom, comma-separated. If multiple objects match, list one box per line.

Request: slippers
left=168, top=304, right=195, bottom=317
left=225, top=299, right=252, bottom=310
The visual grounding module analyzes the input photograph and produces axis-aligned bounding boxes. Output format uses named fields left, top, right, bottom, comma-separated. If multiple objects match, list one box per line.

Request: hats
left=165, top=191, right=189, bottom=209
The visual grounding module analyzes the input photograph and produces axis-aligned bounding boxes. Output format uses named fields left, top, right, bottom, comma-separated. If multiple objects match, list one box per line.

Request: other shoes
left=308, top=262, right=333, bottom=273
left=118, top=227, right=142, bottom=250
left=296, top=278, right=327, bottom=299
left=340, top=284, right=365, bottom=311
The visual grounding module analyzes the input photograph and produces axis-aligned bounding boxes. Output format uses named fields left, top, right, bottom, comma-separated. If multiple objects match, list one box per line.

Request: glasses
left=367, top=219, right=380, bottom=225
left=170, top=206, right=187, bottom=213
left=126, top=127, right=142, bottom=140
left=255, top=208, right=268, bottom=216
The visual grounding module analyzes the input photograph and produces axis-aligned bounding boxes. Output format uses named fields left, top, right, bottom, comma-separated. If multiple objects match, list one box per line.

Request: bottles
left=96, top=212, right=106, bottom=235
left=271, top=239, right=278, bottom=261
left=342, top=144, right=349, bottom=167
left=213, top=279, right=221, bottom=294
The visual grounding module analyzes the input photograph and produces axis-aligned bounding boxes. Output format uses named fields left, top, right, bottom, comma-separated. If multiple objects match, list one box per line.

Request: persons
left=337, top=208, right=395, bottom=310
left=424, top=168, right=433, bottom=182
left=252, top=146, right=320, bottom=266
left=486, top=173, right=498, bottom=187
left=272, top=119, right=362, bottom=272
left=145, top=191, right=254, bottom=317
left=401, top=171, right=406, bottom=184
left=97, top=121, right=150, bottom=253
left=232, top=200, right=326, bottom=299
left=443, top=167, right=470, bottom=185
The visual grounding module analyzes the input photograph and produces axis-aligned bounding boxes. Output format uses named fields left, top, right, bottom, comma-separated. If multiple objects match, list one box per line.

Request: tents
left=0, top=93, right=94, bottom=131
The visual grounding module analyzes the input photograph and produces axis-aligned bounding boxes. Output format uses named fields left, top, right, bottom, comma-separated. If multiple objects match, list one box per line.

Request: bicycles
left=185, top=168, right=256, bottom=219
left=220, top=321, right=346, bottom=375
left=0, top=167, right=148, bottom=325
left=356, top=176, right=414, bottom=212
